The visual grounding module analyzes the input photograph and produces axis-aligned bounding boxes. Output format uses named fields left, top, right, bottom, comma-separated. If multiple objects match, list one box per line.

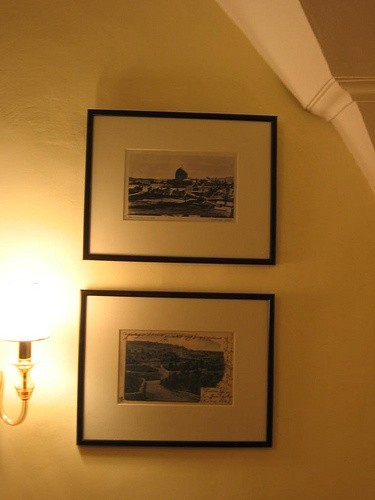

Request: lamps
left=0, top=263, right=52, bottom=425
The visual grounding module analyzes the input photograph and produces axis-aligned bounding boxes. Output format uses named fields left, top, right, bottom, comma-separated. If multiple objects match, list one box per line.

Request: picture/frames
left=82, top=108, right=278, bottom=265
left=76, top=289, right=276, bottom=448
